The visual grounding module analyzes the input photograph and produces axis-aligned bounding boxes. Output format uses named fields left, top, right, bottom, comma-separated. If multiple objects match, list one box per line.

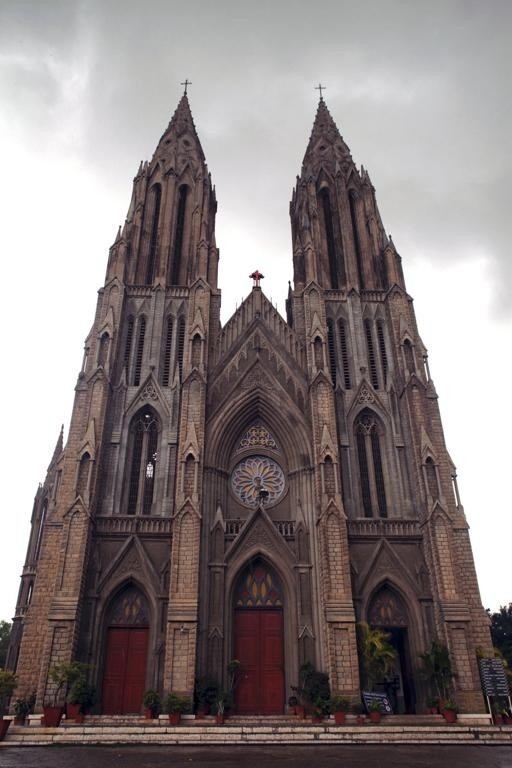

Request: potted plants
left=143, top=659, right=242, bottom=726
left=0, top=660, right=97, bottom=743
left=286, top=663, right=382, bottom=724
left=416, top=638, right=458, bottom=724
left=492, top=701, right=512, bottom=724
